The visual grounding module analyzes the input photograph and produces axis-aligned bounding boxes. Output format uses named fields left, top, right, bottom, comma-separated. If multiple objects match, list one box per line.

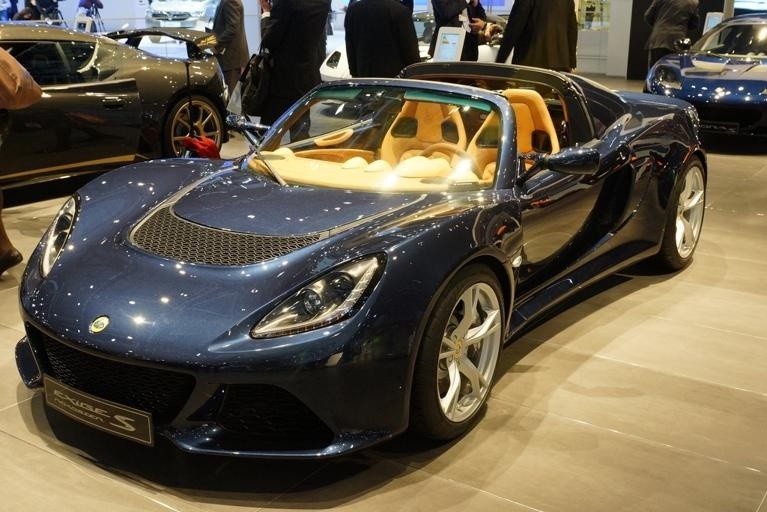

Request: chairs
left=379, top=88, right=562, bottom=180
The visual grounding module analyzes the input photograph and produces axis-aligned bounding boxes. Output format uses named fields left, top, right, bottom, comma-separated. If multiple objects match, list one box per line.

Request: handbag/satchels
left=225, top=42, right=277, bottom=120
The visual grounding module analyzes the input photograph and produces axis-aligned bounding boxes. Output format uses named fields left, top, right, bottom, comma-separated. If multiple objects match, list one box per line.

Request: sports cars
left=14, top=60, right=708, bottom=461
left=1, top=22, right=229, bottom=191
left=644, top=11, right=766, bottom=146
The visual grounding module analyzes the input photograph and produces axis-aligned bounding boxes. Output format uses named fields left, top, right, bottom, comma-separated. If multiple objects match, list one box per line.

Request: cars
left=145, top=1, right=218, bottom=44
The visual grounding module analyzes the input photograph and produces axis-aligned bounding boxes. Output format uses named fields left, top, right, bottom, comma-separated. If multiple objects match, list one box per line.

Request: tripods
left=89, top=6, right=108, bottom=34
left=50, top=4, right=69, bottom=29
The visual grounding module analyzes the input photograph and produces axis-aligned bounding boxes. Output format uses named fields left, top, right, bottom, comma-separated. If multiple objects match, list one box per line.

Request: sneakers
left=0, top=245, right=24, bottom=276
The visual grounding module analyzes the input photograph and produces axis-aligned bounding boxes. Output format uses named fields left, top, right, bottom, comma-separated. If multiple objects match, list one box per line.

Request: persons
left=212, top=0, right=251, bottom=142
left=12, top=0, right=105, bottom=33
left=428, top=1, right=504, bottom=62
left=495, top=0, right=576, bottom=73
left=254, top=1, right=331, bottom=140
left=644, top=0, right=700, bottom=73
left=0, top=214, right=22, bottom=276
left=343, top=1, right=421, bottom=81
